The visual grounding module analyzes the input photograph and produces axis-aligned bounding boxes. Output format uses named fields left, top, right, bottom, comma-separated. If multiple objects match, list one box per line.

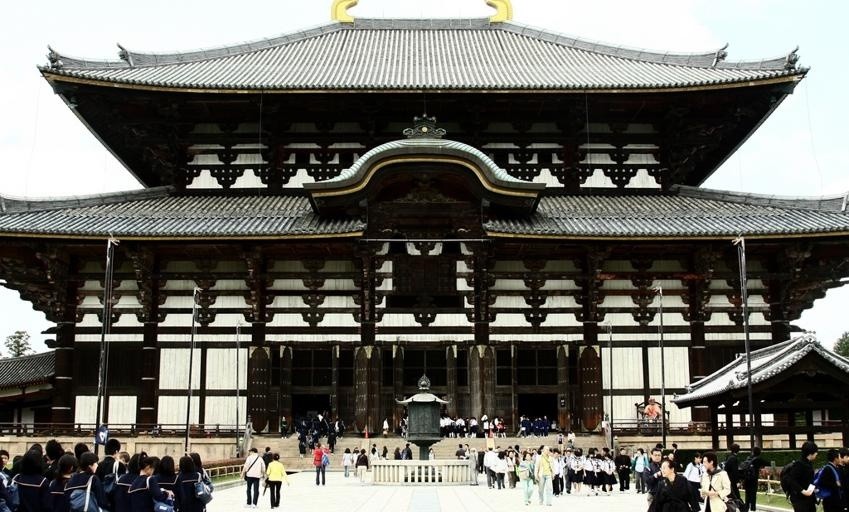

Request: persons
left=241, top=448, right=266, bottom=507
left=0, top=438, right=213, bottom=511
left=265, top=454, right=291, bottom=508
left=643, top=398, right=661, bottom=429
left=280, top=410, right=412, bottom=485
left=263, top=447, right=273, bottom=488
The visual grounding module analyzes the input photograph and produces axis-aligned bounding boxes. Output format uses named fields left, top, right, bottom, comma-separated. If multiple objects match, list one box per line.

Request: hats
left=249, top=448, right=258, bottom=451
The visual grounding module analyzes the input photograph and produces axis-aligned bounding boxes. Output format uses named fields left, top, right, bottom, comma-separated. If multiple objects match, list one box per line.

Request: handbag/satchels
left=5, top=482, right=21, bottom=507
left=70, top=480, right=108, bottom=512
left=153, top=495, right=174, bottom=512
left=518, top=467, right=529, bottom=480
left=726, top=498, right=747, bottom=512
left=244, top=471, right=247, bottom=480
left=103, top=474, right=121, bottom=493
left=194, top=468, right=213, bottom=505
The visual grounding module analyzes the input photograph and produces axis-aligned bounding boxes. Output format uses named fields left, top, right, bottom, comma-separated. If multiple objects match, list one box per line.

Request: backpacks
left=738, top=455, right=761, bottom=482
left=815, top=464, right=840, bottom=499
left=321, top=450, right=330, bottom=470
left=781, top=461, right=805, bottom=497
